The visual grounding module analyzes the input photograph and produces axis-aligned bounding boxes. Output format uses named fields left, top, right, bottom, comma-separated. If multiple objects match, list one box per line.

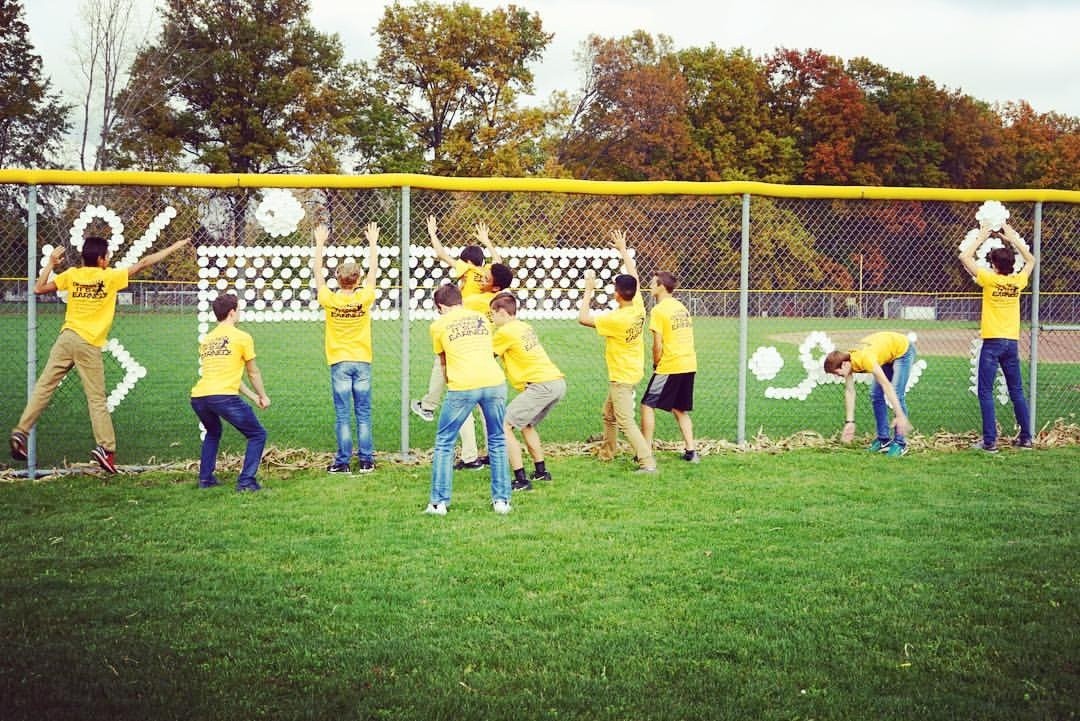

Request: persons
left=190, top=293, right=271, bottom=493
left=579, top=229, right=657, bottom=473
left=313, top=222, right=382, bottom=475
left=958, top=223, right=1036, bottom=454
left=410, top=215, right=567, bottom=515
left=8, top=237, right=191, bottom=474
left=627, top=272, right=699, bottom=469
left=823, top=331, right=917, bottom=458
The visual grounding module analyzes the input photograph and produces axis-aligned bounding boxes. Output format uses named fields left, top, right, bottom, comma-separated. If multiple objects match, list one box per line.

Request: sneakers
left=91, top=445, right=118, bottom=475
left=359, top=460, right=376, bottom=473
left=411, top=398, right=435, bottom=421
left=8, top=433, right=29, bottom=460
left=326, top=462, right=352, bottom=475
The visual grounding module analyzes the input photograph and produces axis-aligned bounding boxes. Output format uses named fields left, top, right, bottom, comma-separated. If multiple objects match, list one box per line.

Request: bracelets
left=845, top=420, right=855, bottom=424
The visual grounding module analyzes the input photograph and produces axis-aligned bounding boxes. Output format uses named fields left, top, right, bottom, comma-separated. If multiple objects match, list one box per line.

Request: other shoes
left=632, top=456, right=639, bottom=465
left=981, top=441, right=997, bottom=453
left=681, top=448, right=699, bottom=463
left=198, top=478, right=221, bottom=489
left=886, top=440, right=909, bottom=457
left=864, top=439, right=893, bottom=453
left=479, top=455, right=490, bottom=465
left=494, top=501, right=511, bottom=514
left=511, top=479, right=530, bottom=492
left=421, top=502, right=447, bottom=514
left=453, top=459, right=483, bottom=471
left=528, top=471, right=553, bottom=481
left=1010, top=438, right=1033, bottom=449
